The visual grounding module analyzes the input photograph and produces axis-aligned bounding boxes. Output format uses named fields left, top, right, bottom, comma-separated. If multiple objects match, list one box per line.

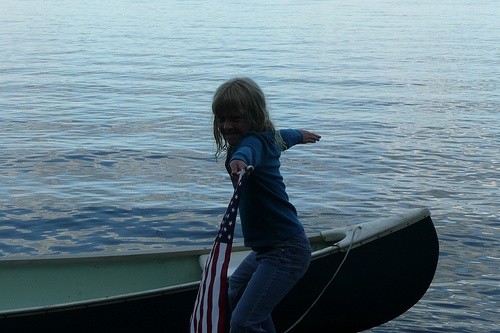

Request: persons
left=211, top=77, right=321, bottom=333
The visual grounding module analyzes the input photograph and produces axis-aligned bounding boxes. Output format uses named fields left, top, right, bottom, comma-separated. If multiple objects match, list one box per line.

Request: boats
left=0, top=208, right=439, bottom=333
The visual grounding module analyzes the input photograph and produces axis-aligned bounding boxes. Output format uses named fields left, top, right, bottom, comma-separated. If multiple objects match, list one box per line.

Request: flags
left=190, top=168, right=248, bottom=332
